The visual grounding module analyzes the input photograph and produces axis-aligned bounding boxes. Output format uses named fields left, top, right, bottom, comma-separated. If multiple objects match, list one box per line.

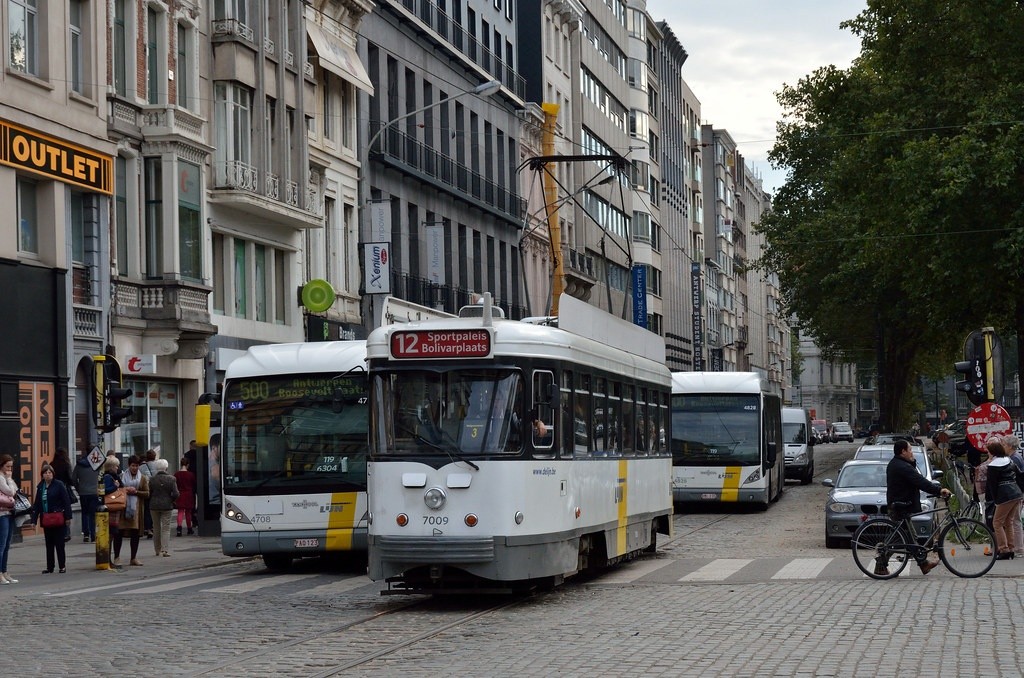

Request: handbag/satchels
left=42, top=510, right=65, bottom=527
left=103, top=474, right=127, bottom=511
left=0, top=489, right=34, bottom=517
left=66, top=482, right=78, bottom=504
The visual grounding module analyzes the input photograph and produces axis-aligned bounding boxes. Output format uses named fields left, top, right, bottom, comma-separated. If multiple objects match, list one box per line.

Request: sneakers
left=0, top=572, right=19, bottom=585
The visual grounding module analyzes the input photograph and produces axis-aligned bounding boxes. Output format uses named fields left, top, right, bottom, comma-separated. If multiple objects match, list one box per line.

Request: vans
left=781, top=407, right=815, bottom=484
left=832, top=424, right=854, bottom=443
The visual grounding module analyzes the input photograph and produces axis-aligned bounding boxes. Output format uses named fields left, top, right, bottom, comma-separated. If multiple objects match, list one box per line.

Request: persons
left=74, top=445, right=98, bottom=542
left=30, top=464, right=73, bottom=574
left=0, top=454, right=20, bottom=585
left=209, top=434, right=221, bottom=504
left=49, top=447, right=73, bottom=542
left=81, top=440, right=196, bottom=566
left=911, top=422, right=1024, bottom=560
left=476, top=389, right=547, bottom=454
left=874, top=439, right=951, bottom=575
left=517, top=378, right=668, bottom=455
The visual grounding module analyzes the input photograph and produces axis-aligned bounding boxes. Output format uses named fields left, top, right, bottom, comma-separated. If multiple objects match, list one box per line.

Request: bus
left=333, top=294, right=674, bottom=596
left=669, top=372, right=784, bottom=511
left=194, top=341, right=369, bottom=570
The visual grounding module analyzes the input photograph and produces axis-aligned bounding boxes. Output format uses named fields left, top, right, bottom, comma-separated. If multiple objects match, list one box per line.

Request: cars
left=933, top=419, right=969, bottom=445
left=822, top=459, right=940, bottom=549
left=851, top=434, right=942, bottom=483
left=812, top=427, right=829, bottom=444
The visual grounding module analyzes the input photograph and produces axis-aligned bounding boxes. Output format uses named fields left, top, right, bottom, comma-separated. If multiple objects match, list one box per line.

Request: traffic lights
left=954, top=337, right=984, bottom=402
left=106, top=379, right=133, bottom=427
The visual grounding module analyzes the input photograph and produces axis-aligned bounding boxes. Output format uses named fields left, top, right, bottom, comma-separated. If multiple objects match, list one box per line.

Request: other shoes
left=114, top=557, right=122, bottom=565
left=59, top=567, right=67, bottom=573
left=984, top=551, right=993, bottom=556
left=65, top=535, right=71, bottom=542
left=176, top=529, right=182, bottom=536
left=192, top=512, right=199, bottom=528
left=1014, top=549, right=1024, bottom=558
left=144, top=529, right=154, bottom=539
left=156, top=551, right=171, bottom=557
left=42, top=568, right=54, bottom=573
left=83, top=536, right=96, bottom=544
left=187, top=529, right=194, bottom=534
left=110, top=563, right=122, bottom=569
left=130, top=558, right=144, bottom=566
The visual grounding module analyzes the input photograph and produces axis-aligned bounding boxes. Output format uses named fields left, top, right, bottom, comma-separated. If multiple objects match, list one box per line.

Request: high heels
left=995, top=551, right=1015, bottom=560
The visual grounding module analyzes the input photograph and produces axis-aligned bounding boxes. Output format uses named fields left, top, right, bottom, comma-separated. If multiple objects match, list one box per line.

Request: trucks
left=811, top=420, right=826, bottom=435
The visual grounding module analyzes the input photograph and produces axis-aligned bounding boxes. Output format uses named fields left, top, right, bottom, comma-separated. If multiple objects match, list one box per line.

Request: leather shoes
left=873, top=567, right=890, bottom=575
left=920, top=557, right=941, bottom=575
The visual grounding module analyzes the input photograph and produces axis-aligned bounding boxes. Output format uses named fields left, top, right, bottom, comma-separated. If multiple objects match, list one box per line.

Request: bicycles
left=850, top=492, right=997, bottom=579
left=955, top=484, right=982, bottom=543
left=945, top=454, right=967, bottom=491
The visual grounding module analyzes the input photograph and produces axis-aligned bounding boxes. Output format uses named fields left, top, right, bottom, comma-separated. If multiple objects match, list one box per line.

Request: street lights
left=361, top=80, right=501, bottom=343
